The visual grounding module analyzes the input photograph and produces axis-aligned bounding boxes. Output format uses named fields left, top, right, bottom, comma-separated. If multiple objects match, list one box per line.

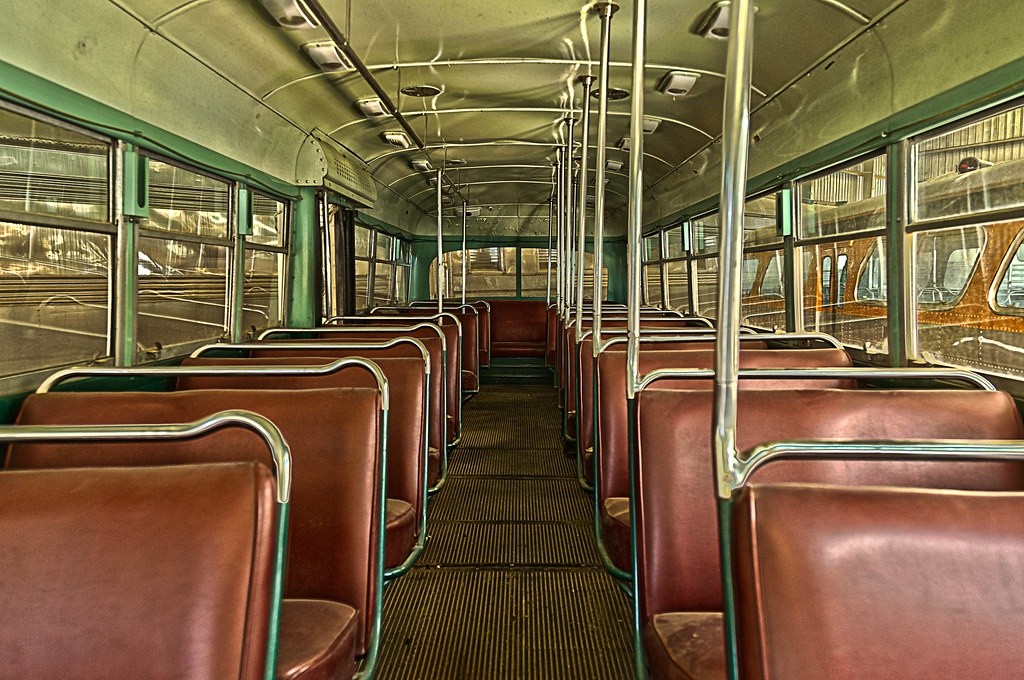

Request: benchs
left=0, top=298, right=1024, bottom=680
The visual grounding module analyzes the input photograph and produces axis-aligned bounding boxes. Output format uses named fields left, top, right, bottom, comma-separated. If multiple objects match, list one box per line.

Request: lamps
left=259, top=0, right=321, bottom=31
left=605, top=160, right=624, bottom=172
left=442, top=194, right=456, bottom=206
left=587, top=194, right=595, bottom=203
left=383, top=129, right=412, bottom=150
left=691, top=0, right=760, bottom=42
left=457, top=207, right=483, bottom=218
left=618, top=139, right=645, bottom=153
left=308, top=43, right=352, bottom=77
left=353, top=96, right=391, bottom=121
left=429, top=177, right=437, bottom=188
left=605, top=178, right=610, bottom=184
left=656, top=68, right=703, bottom=101
left=644, top=117, right=663, bottom=135
left=413, top=160, right=433, bottom=172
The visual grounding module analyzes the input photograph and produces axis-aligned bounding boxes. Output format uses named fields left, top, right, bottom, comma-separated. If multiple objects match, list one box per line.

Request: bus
left=1, top=98, right=401, bottom=398
left=700, top=157, right=1023, bottom=415
